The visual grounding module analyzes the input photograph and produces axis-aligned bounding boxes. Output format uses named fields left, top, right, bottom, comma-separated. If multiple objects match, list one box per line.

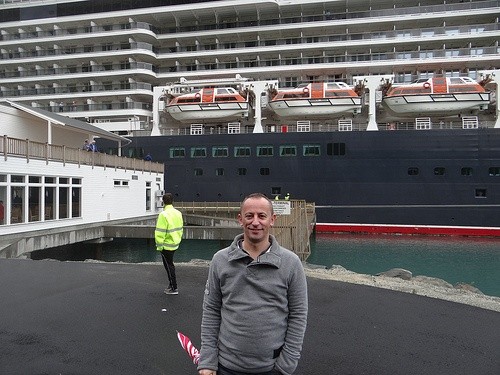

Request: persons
left=82, top=140, right=98, bottom=152
left=143, top=152, right=154, bottom=162
left=0, top=200, right=5, bottom=225
left=197, top=194, right=308, bottom=375
left=285, top=192, right=292, bottom=201
left=154, top=193, right=184, bottom=294
left=274, top=194, right=281, bottom=201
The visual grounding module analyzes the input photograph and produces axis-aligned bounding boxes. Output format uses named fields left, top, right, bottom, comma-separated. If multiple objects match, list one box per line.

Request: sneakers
left=164, top=288, right=169, bottom=293
left=166, top=289, right=178, bottom=294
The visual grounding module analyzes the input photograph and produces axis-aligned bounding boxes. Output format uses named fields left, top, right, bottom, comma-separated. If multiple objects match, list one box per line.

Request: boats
left=268, top=82, right=362, bottom=118
left=381, top=77, right=491, bottom=118
left=165, top=87, right=248, bottom=123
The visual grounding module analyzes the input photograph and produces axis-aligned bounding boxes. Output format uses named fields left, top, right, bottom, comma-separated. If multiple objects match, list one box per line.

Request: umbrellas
left=176, top=330, right=200, bottom=366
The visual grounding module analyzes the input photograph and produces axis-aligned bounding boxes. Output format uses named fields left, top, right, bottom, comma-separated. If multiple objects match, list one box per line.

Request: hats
left=92, top=140, right=96, bottom=142
